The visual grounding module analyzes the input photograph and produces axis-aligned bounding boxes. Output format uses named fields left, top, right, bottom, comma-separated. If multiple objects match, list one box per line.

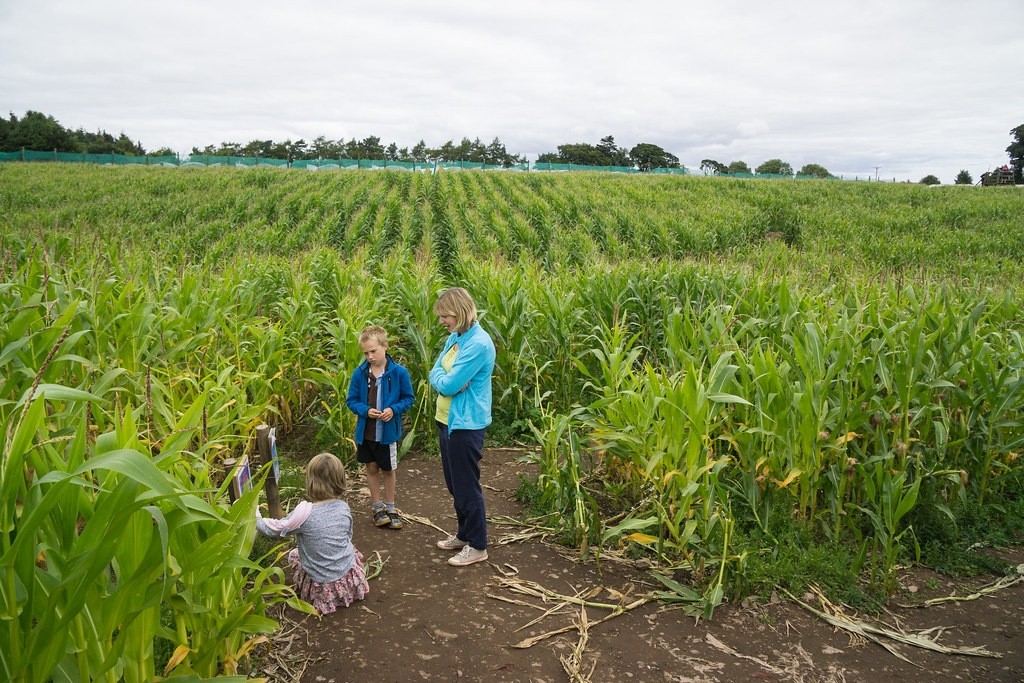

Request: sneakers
left=436, top=535, right=470, bottom=549
left=448, top=543, right=489, bottom=566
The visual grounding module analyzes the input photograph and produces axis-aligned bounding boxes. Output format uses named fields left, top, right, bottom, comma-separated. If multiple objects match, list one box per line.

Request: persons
left=255, top=452, right=369, bottom=614
left=427, top=286, right=496, bottom=566
left=346, top=324, right=415, bottom=529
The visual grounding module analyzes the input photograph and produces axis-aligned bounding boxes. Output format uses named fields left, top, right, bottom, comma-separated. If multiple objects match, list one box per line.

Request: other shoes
left=372, top=509, right=391, bottom=526
left=385, top=508, right=402, bottom=528
